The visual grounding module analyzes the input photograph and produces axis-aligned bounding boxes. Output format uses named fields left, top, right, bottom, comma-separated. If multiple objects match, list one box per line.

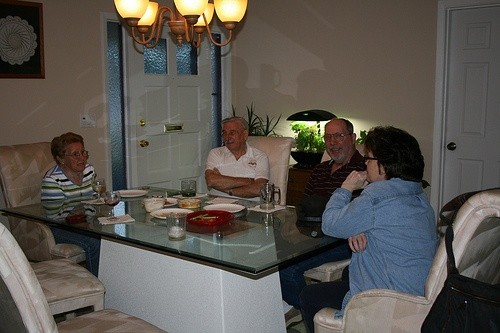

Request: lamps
left=113, top=0, right=249, bottom=48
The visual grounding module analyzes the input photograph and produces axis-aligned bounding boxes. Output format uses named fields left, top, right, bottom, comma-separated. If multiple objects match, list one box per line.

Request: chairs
left=313, top=190, right=500, bottom=333
left=246, top=136, right=294, bottom=206
left=0, top=142, right=167, bottom=333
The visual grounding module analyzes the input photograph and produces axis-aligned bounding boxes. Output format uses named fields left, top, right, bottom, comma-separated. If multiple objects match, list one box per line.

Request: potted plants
left=289, top=122, right=327, bottom=167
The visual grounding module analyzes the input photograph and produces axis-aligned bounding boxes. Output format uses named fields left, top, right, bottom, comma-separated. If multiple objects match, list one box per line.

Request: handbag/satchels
left=421, top=273, right=500, bottom=333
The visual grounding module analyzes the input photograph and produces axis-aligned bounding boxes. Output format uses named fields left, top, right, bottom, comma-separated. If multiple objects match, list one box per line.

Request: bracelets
left=229, top=189, right=232, bottom=196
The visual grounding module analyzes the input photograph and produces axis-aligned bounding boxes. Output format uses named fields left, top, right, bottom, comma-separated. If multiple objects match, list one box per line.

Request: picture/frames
left=0, top=0, right=45, bottom=80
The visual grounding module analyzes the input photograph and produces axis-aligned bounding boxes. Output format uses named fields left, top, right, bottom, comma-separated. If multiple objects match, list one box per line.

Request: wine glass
left=92, top=177, right=106, bottom=203
left=105, top=191, right=121, bottom=221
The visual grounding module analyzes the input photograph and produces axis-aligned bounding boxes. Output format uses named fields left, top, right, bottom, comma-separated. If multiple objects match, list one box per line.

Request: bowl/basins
left=145, top=198, right=163, bottom=212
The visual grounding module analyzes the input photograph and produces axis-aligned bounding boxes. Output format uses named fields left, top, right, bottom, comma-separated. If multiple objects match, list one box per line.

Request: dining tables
left=0, top=187, right=350, bottom=333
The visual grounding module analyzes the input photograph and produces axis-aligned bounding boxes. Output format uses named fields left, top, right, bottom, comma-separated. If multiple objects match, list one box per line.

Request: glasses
left=323, top=133, right=351, bottom=141
left=58, top=150, right=88, bottom=159
left=362, top=155, right=378, bottom=162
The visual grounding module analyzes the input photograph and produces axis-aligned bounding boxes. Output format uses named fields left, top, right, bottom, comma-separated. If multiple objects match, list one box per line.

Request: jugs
left=259, top=179, right=281, bottom=210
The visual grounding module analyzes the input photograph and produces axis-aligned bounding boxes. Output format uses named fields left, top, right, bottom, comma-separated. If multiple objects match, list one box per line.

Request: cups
left=181, top=180, right=197, bottom=197
left=166, top=214, right=186, bottom=241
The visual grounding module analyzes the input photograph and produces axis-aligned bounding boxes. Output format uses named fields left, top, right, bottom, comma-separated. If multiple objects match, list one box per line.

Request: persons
left=299, top=125, right=437, bottom=333
left=204, top=117, right=269, bottom=201
left=41, top=132, right=106, bottom=277
left=280, top=119, right=367, bottom=311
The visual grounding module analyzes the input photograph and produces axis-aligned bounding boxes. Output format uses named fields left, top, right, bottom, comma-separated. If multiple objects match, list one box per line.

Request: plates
left=112, top=190, right=148, bottom=197
left=163, top=197, right=177, bottom=206
left=186, top=210, right=235, bottom=226
left=150, top=208, right=193, bottom=219
left=203, top=204, right=244, bottom=213
